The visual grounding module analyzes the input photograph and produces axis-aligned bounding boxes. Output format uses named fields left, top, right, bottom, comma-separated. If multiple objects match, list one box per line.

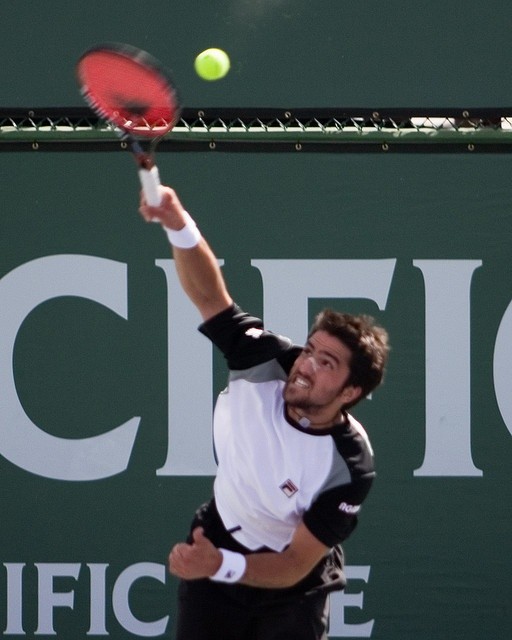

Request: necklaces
left=291, top=407, right=341, bottom=428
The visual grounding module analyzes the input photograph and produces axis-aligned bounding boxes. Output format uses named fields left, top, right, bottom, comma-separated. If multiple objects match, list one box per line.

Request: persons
left=137, top=185, right=389, bottom=634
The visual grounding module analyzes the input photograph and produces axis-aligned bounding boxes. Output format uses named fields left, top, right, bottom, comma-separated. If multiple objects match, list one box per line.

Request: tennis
left=194, top=48, right=230, bottom=82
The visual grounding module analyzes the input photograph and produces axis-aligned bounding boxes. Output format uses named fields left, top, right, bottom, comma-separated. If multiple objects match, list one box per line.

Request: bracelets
left=162, top=210, right=201, bottom=249
left=210, top=548, right=247, bottom=585
